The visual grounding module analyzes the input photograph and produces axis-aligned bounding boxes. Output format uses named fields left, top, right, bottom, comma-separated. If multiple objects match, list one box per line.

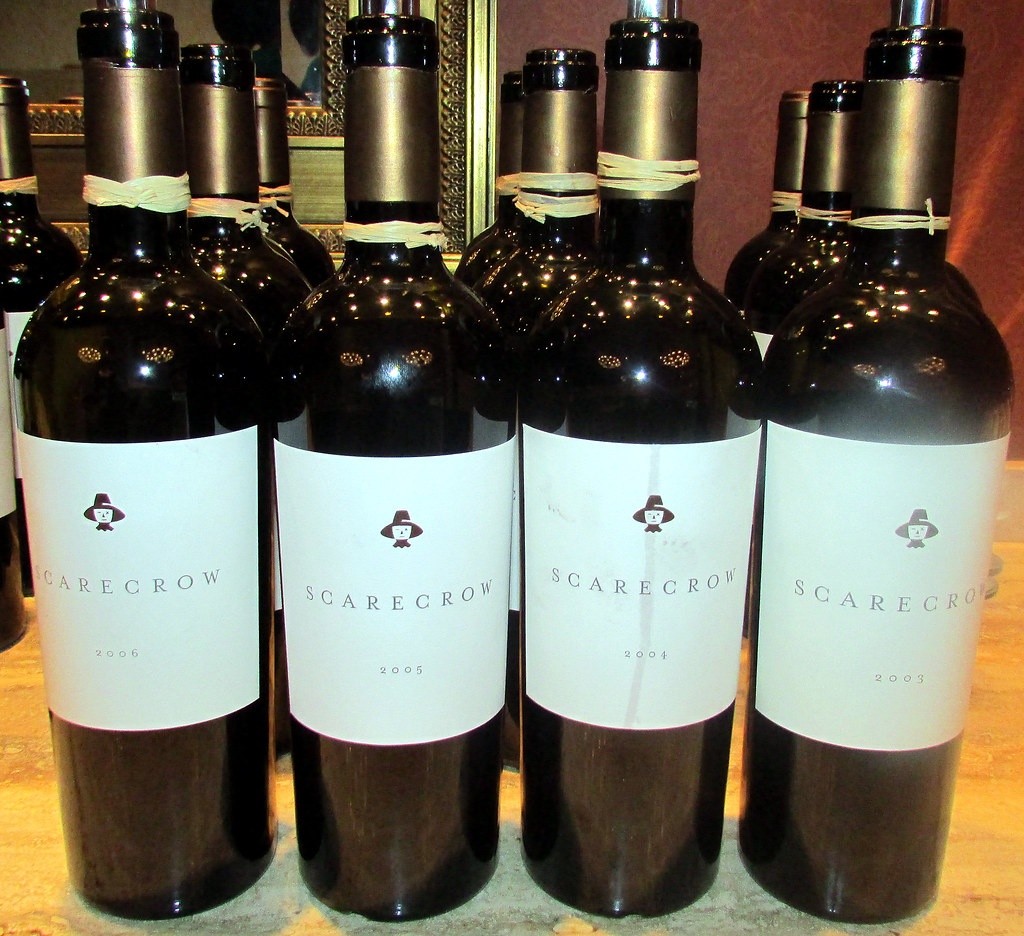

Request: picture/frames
left=0, top=0, right=498, bottom=274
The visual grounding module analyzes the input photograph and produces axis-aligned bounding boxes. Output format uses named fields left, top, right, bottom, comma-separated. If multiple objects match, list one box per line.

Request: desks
left=0, top=461, right=1024, bottom=936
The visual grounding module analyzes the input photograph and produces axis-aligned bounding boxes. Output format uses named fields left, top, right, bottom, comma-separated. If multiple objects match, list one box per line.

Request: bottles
left=270, top=13, right=509, bottom=927
left=521, top=23, right=760, bottom=917
left=737, top=23, right=1006, bottom=921
left=14, top=5, right=285, bottom=919
left=0, top=45, right=856, bottom=763
